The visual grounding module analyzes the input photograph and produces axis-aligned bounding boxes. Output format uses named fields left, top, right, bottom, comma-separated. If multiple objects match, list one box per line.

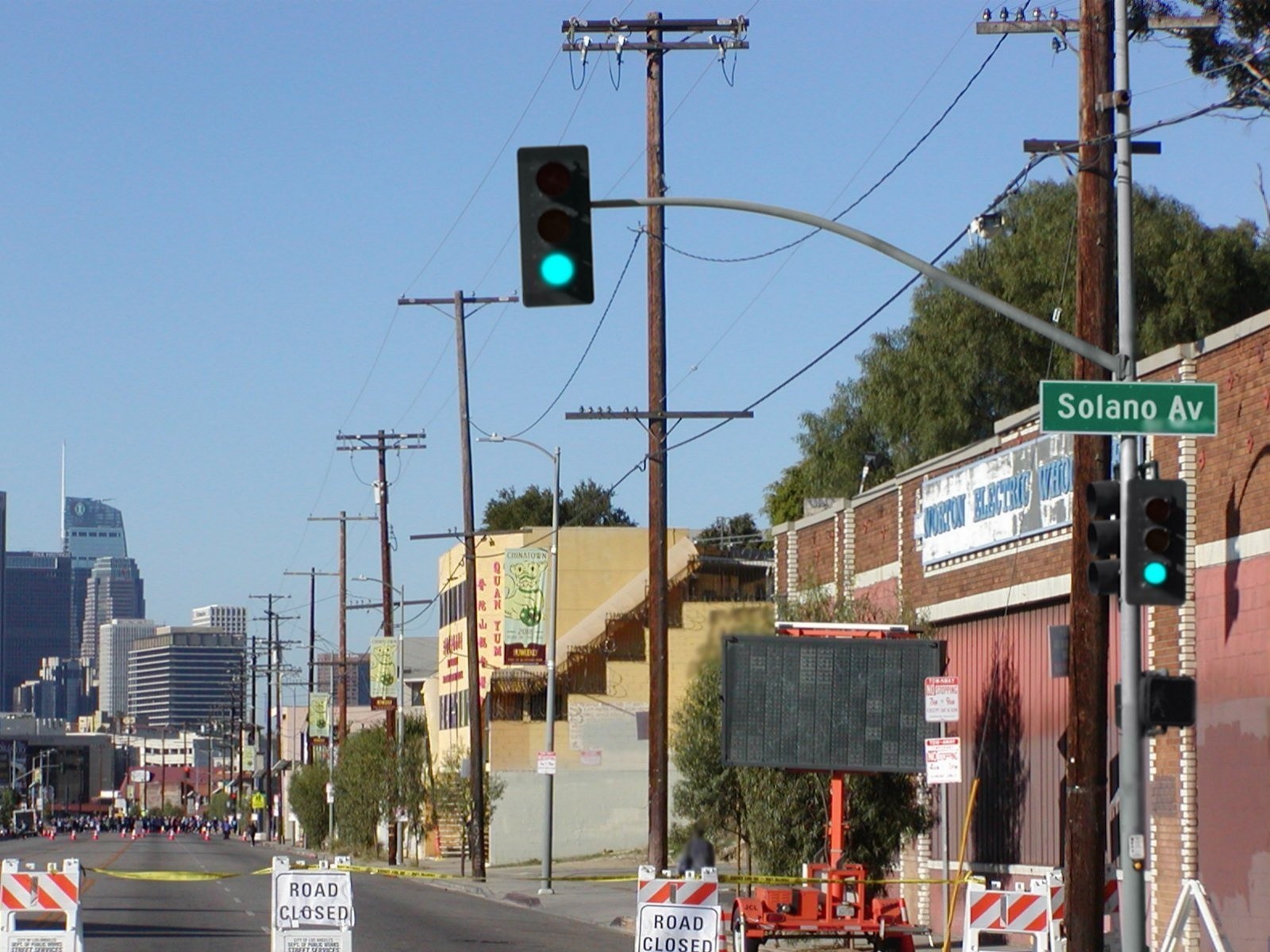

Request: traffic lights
left=1125, top=480, right=1186, bottom=606
left=1084, top=478, right=1122, bottom=599
left=183, top=766, right=191, bottom=778
left=1140, top=675, right=1197, bottom=728
left=517, top=144, right=594, bottom=307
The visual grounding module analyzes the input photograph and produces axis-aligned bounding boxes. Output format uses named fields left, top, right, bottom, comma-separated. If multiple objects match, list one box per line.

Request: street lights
left=296, top=644, right=335, bottom=843
left=237, top=716, right=261, bottom=834
left=473, top=434, right=559, bottom=891
left=351, top=574, right=405, bottom=867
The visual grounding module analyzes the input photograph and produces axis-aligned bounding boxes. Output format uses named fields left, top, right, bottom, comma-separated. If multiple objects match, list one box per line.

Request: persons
left=3, top=812, right=258, bottom=848
left=676, top=821, right=715, bottom=876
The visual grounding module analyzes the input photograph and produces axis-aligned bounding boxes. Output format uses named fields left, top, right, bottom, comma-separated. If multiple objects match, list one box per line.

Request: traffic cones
left=202, top=825, right=210, bottom=841
left=70, top=829, right=77, bottom=840
left=42, top=826, right=55, bottom=840
left=168, top=830, right=175, bottom=840
left=130, top=826, right=151, bottom=839
left=177, top=825, right=181, bottom=833
left=160, top=825, right=165, bottom=835
left=92, top=830, right=99, bottom=840
left=120, top=828, right=126, bottom=839
